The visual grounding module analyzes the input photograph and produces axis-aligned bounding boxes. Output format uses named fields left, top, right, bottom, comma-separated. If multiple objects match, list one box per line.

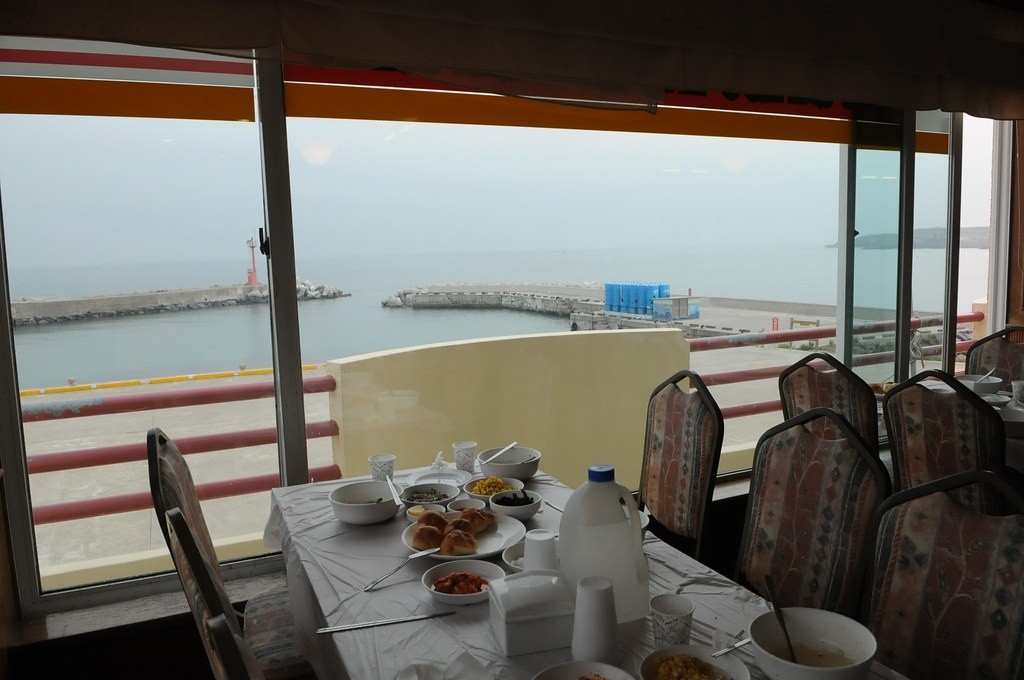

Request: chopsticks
left=711, top=637, right=753, bottom=659
left=316, top=611, right=456, bottom=633
left=481, top=441, right=518, bottom=465
left=386, top=474, right=403, bottom=505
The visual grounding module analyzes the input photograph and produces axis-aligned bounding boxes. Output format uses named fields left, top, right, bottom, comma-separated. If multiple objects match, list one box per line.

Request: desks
left=877, top=379, right=1024, bottom=428
left=263, top=458, right=910, bottom=680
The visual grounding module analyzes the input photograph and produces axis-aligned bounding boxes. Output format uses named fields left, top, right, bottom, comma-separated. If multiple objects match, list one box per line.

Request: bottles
left=559, top=464, right=651, bottom=624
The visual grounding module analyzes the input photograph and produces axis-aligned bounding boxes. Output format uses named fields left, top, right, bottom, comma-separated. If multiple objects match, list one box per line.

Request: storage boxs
left=604, top=282, right=698, bottom=317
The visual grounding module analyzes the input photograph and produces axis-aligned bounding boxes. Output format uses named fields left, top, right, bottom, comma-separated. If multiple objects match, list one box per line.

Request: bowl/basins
left=328, top=481, right=405, bottom=525
left=400, top=482, right=486, bottom=523
left=489, top=490, right=542, bottom=522
left=953, top=374, right=1004, bottom=394
left=748, top=607, right=877, bottom=680
left=622, top=505, right=650, bottom=542
left=477, top=447, right=541, bottom=481
left=640, top=645, right=750, bottom=680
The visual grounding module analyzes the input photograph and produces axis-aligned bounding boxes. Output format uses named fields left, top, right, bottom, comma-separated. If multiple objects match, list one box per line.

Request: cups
left=650, top=594, right=696, bottom=650
left=523, top=529, right=558, bottom=571
left=452, top=440, right=478, bottom=472
left=368, top=454, right=396, bottom=481
left=1012, top=381, right=1024, bottom=403
left=571, top=576, right=621, bottom=666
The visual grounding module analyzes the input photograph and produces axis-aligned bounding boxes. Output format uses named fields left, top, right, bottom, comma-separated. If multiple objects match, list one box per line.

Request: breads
left=412, top=507, right=495, bottom=556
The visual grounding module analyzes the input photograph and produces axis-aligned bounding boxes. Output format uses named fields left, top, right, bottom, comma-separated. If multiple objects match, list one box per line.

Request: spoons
left=363, top=547, right=441, bottom=591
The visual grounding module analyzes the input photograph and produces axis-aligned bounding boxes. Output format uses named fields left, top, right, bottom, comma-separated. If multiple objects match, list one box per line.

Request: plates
left=531, top=661, right=636, bottom=680
left=401, top=511, right=526, bottom=561
left=871, top=383, right=901, bottom=399
left=981, top=393, right=1012, bottom=406
left=407, top=468, right=472, bottom=487
left=463, top=477, right=524, bottom=504
left=422, top=560, right=506, bottom=604
left=502, top=540, right=525, bottom=572
left=917, top=380, right=958, bottom=395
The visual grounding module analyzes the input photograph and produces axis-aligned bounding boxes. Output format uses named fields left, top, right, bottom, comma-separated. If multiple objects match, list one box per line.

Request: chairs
left=641, top=326, right=1024, bottom=680
left=149, top=430, right=317, bottom=680
